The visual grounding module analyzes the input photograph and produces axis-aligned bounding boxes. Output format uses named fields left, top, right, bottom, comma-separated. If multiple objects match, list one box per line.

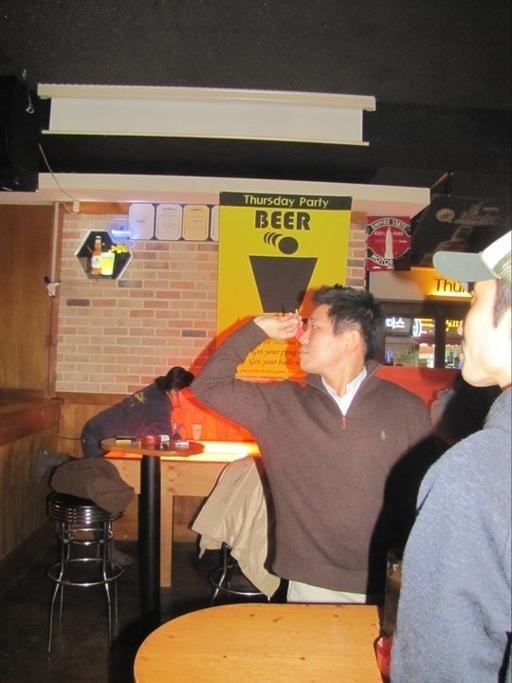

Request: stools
left=43, top=488, right=126, bottom=656
left=207, top=455, right=284, bottom=603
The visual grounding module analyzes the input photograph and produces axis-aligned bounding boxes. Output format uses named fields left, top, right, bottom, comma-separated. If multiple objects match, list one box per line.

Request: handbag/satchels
left=48, top=455, right=136, bottom=515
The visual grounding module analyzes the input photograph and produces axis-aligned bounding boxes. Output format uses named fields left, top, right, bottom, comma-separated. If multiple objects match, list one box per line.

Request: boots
left=96, top=538, right=135, bottom=567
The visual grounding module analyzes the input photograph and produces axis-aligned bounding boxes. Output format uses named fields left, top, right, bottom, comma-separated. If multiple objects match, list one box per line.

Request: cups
left=191, top=424, right=203, bottom=442
left=99, top=251, right=116, bottom=276
left=373, top=635, right=392, bottom=681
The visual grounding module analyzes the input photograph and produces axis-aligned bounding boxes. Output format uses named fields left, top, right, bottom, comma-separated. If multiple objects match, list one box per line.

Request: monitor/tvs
left=0, top=74, right=40, bottom=192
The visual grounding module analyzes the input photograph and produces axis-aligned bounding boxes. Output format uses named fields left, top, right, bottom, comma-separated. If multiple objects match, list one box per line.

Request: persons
left=387, top=227, right=511, bottom=683
left=189, top=283, right=443, bottom=604
left=80, top=366, right=195, bottom=459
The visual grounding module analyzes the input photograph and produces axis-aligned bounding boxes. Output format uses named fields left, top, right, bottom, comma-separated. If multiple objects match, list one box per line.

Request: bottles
left=90, top=235, right=104, bottom=275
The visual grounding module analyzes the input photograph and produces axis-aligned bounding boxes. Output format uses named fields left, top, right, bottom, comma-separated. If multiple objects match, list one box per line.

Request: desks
left=99, top=435, right=261, bottom=641
left=132, top=599, right=386, bottom=683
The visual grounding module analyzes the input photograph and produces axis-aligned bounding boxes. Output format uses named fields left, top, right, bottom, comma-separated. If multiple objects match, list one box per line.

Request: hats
left=431, top=227, right=512, bottom=294
